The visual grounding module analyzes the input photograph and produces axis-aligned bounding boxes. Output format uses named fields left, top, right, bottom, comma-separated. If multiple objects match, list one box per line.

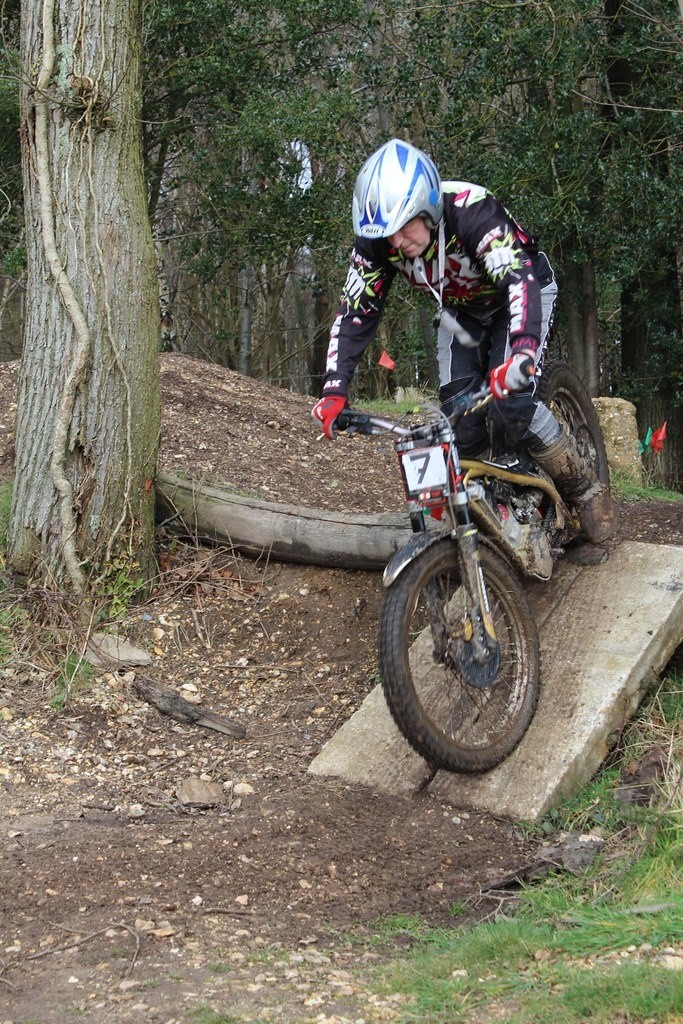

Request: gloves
left=311, top=395, right=352, bottom=440
left=490, top=349, right=538, bottom=401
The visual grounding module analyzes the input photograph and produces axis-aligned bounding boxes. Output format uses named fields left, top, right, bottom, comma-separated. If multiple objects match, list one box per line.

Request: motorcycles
left=332, top=360, right=614, bottom=777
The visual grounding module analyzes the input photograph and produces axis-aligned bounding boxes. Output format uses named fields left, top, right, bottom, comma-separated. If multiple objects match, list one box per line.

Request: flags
left=374, top=350, right=396, bottom=375
left=636, top=419, right=669, bottom=456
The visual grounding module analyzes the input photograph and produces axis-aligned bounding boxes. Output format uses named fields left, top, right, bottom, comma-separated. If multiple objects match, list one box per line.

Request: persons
left=309, top=135, right=618, bottom=544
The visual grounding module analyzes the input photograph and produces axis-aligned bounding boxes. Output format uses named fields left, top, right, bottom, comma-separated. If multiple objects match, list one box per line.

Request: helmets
left=351, top=138, right=444, bottom=238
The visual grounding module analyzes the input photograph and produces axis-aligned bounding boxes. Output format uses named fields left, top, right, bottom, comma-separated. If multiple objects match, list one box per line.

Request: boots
left=526, top=417, right=616, bottom=543
